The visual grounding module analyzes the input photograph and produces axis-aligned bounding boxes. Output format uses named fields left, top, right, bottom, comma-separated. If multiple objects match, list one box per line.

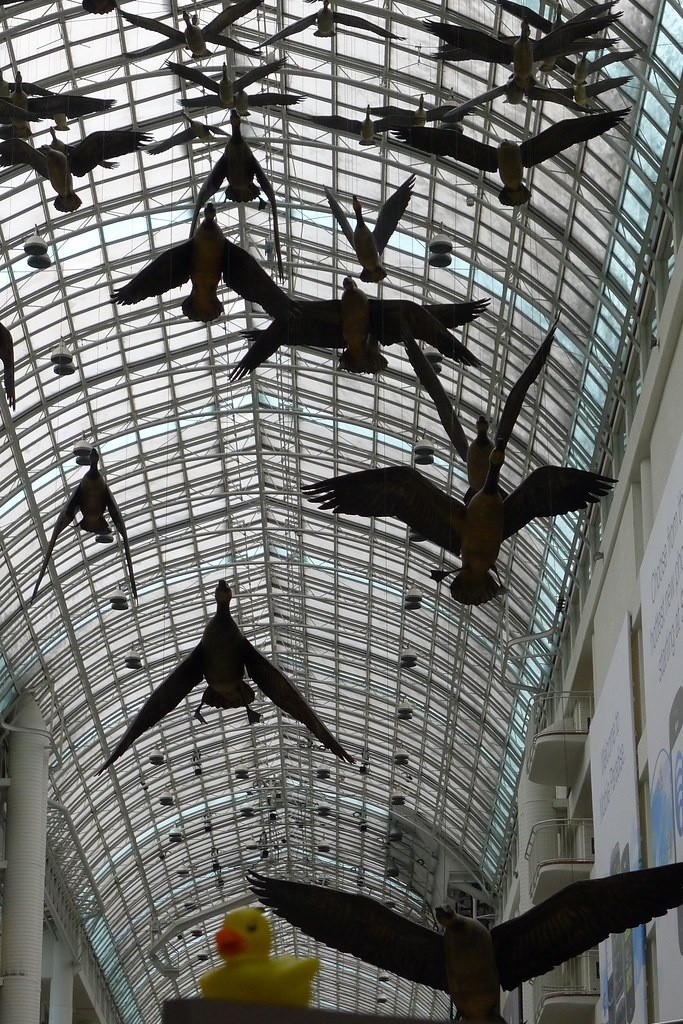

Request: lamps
left=148, top=746, right=408, bottom=1006
left=414, top=437, right=434, bottom=464
left=404, top=579, right=422, bottom=610
left=427, top=233, right=453, bottom=268
left=400, top=646, right=418, bottom=669
left=75, top=432, right=95, bottom=466
left=51, top=337, right=76, bottom=375
left=24, top=222, right=52, bottom=267
left=110, top=585, right=129, bottom=609
left=125, top=647, right=144, bottom=669
left=422, top=343, right=443, bottom=372
left=437, top=93, right=466, bottom=134
left=96, top=533, right=113, bottom=543
left=398, top=697, right=415, bottom=720
left=410, top=529, right=426, bottom=541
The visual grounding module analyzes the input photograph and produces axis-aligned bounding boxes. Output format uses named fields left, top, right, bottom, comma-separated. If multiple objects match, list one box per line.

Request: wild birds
left=31, top=447, right=141, bottom=603
left=0, top=69, right=154, bottom=214
left=322, top=173, right=417, bottom=282
left=244, top=861, right=683, bottom=1024
left=95, top=579, right=354, bottom=774
left=176, top=88, right=307, bottom=116
left=110, top=200, right=292, bottom=321
left=252, top=0, right=407, bottom=49
left=146, top=111, right=230, bottom=156
left=82, top=0, right=263, bottom=59
left=230, top=273, right=491, bottom=376
left=309, top=104, right=425, bottom=146
left=395, top=0, right=643, bottom=207
left=403, top=314, right=560, bottom=499
left=304, top=431, right=620, bottom=608
left=187, top=107, right=284, bottom=275
left=364, top=94, right=475, bottom=126
left=0, top=322, right=15, bottom=411
left=164, top=56, right=288, bottom=105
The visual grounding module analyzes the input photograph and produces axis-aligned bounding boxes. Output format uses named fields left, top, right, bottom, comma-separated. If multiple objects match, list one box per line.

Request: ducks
left=197, top=908, right=318, bottom=1008
